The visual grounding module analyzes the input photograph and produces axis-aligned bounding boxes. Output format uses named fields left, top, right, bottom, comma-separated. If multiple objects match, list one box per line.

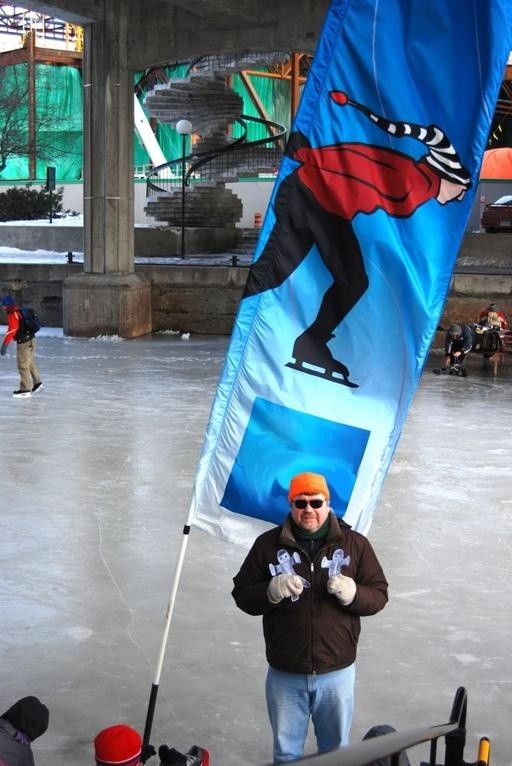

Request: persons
left=232, top=473, right=389, bottom=763
left=242, top=91, right=472, bottom=376
left=0, top=296, right=43, bottom=398
left=480, top=304, right=508, bottom=358
left=0, top=696, right=50, bottom=766
left=443, top=320, right=476, bottom=372
left=94, top=725, right=210, bottom=766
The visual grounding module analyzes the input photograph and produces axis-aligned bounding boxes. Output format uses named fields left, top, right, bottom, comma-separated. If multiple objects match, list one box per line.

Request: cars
left=481, top=194, right=512, bottom=233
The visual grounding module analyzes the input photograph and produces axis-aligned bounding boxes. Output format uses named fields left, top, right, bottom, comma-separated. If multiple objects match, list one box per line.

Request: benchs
left=468, top=333, right=512, bottom=376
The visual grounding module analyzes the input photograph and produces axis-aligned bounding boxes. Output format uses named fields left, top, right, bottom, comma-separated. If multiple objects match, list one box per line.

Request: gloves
left=0, top=345, right=7, bottom=356
left=326, top=575, right=356, bottom=607
left=267, top=574, right=303, bottom=604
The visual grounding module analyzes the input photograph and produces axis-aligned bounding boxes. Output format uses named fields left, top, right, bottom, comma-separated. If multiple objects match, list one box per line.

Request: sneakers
left=13, top=382, right=44, bottom=396
left=431, top=364, right=468, bottom=376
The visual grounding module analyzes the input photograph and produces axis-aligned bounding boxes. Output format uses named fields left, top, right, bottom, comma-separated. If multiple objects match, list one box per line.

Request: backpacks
left=15, top=306, right=44, bottom=335
left=479, top=329, right=502, bottom=353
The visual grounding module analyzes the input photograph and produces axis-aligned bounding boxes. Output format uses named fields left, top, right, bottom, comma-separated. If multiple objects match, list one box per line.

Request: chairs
left=134, top=163, right=153, bottom=180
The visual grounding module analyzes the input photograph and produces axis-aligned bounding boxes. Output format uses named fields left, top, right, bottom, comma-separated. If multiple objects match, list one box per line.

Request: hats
left=288, top=472, right=330, bottom=504
left=94, top=725, right=143, bottom=766
left=3, top=296, right=14, bottom=305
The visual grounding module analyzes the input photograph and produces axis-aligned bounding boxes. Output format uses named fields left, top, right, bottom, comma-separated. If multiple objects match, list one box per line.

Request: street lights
left=175, top=117, right=192, bottom=259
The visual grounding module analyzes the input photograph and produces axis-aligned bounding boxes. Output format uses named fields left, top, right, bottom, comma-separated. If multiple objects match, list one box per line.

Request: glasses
left=294, top=500, right=323, bottom=509
